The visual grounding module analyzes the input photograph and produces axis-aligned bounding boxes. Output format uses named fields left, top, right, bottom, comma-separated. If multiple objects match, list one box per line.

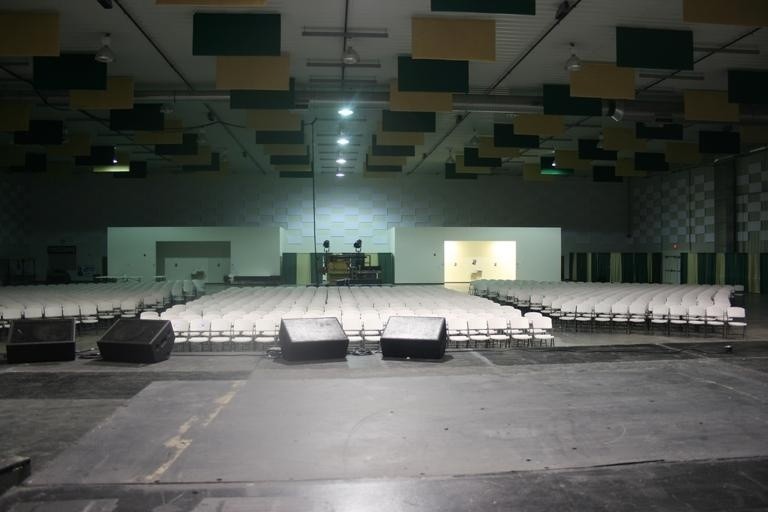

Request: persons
left=469, top=259, right=478, bottom=280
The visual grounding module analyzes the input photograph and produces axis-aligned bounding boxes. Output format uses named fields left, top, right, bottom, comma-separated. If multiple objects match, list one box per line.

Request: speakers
left=5, top=318, right=76, bottom=361
left=277, top=317, right=350, bottom=360
left=380, top=314, right=447, bottom=359
left=96, top=318, right=175, bottom=364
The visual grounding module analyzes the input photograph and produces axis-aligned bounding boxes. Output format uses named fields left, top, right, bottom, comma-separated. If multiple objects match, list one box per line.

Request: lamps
left=342, top=35, right=359, bottom=66
left=94, top=32, right=115, bottom=63
left=563, top=43, right=584, bottom=72
